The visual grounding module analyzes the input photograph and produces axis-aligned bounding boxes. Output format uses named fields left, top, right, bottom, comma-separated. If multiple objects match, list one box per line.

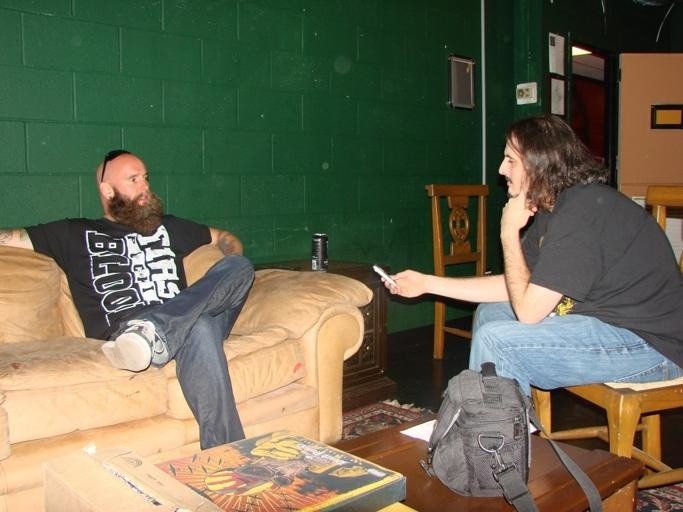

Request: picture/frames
left=651, top=104, right=683, bottom=129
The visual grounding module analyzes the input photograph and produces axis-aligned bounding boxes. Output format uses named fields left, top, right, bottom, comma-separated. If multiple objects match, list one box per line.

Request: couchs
left=0, top=271, right=373, bottom=462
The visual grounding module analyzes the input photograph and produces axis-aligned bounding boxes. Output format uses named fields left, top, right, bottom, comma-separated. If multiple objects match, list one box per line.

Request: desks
left=330, top=412, right=645, bottom=512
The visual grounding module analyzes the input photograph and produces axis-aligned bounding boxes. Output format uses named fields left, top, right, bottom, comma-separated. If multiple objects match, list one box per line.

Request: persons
left=0, top=149, right=256, bottom=452
left=379, top=113, right=683, bottom=398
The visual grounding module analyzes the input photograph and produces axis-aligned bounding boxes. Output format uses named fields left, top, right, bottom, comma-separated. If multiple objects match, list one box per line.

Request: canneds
left=312, top=234, right=328, bottom=272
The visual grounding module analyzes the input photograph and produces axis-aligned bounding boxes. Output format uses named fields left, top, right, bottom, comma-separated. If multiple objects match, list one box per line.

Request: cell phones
left=371, top=263, right=398, bottom=288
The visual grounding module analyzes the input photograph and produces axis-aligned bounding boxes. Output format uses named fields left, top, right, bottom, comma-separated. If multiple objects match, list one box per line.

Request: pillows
left=183, top=242, right=225, bottom=287
left=61, top=271, right=86, bottom=339
left=0, top=243, right=64, bottom=343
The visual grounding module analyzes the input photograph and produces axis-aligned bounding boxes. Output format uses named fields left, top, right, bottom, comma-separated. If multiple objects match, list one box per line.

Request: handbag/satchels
left=419, top=363, right=532, bottom=498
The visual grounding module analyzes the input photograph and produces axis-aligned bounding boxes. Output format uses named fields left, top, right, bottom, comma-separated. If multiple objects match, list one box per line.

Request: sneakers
left=101, top=320, right=164, bottom=372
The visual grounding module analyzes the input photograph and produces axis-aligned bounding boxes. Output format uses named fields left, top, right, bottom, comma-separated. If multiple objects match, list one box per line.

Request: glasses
left=101, top=150, right=130, bottom=183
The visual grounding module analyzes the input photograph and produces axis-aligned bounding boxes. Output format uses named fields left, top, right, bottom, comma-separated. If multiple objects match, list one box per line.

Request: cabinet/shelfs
left=255, top=259, right=396, bottom=412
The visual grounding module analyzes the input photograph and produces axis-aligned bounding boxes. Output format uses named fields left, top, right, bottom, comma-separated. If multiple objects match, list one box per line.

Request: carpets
left=343, top=400, right=683, bottom=511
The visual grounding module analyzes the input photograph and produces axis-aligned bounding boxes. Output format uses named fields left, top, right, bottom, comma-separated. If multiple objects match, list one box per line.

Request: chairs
left=530, top=184, right=683, bottom=489
left=424, top=183, right=489, bottom=361
